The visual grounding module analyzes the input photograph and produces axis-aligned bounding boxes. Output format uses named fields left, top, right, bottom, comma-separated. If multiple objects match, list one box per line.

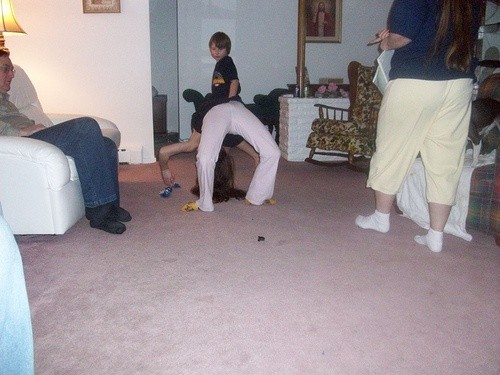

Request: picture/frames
left=304, top=0, right=343, bottom=44
left=82, top=0, right=120, bottom=13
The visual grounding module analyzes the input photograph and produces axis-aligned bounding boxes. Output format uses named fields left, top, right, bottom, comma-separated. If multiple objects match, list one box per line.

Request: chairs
left=304, top=61, right=384, bottom=174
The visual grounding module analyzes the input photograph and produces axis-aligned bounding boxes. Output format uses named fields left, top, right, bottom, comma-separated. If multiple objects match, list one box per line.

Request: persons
left=355, top=0, right=487, bottom=253
left=0, top=47, right=131, bottom=234
left=209, top=32, right=241, bottom=97
left=159, top=96, right=281, bottom=212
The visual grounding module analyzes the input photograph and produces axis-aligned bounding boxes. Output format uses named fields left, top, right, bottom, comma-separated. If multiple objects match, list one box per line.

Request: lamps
left=0, top=0, right=28, bottom=50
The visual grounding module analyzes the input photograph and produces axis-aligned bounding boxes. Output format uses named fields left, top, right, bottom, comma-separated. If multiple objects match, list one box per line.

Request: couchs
left=0, top=63, right=121, bottom=237
left=395, top=59, right=500, bottom=248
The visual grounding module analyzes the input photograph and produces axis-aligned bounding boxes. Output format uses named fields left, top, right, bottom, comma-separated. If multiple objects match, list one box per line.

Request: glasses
left=1, top=66, right=15, bottom=74
